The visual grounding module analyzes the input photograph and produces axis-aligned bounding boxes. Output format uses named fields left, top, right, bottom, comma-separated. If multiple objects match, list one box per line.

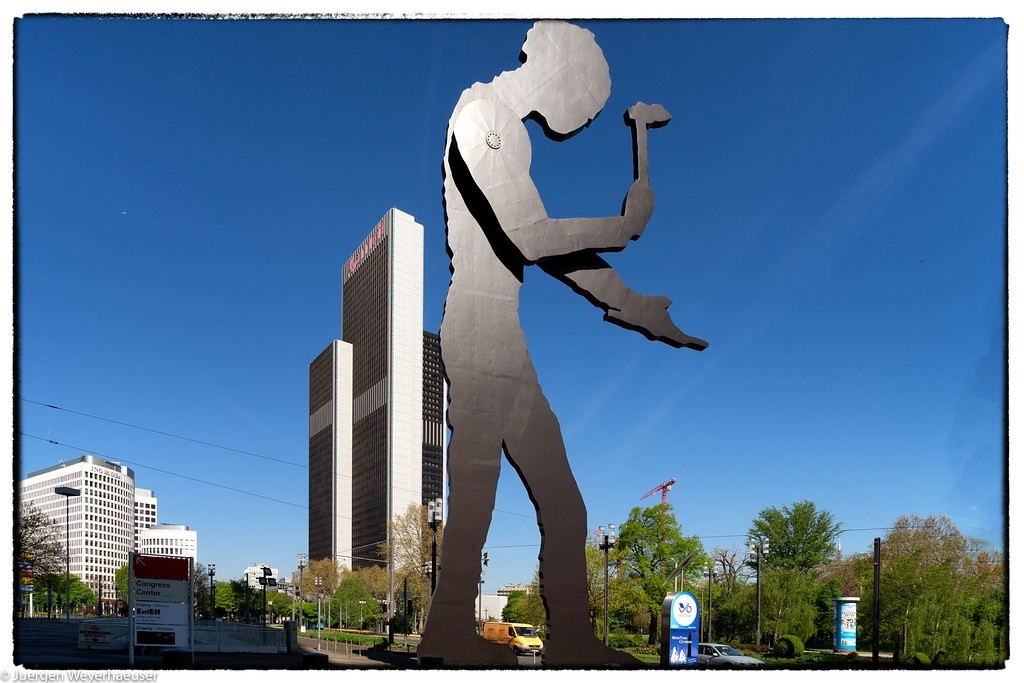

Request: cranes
left=640, top=477, right=677, bottom=577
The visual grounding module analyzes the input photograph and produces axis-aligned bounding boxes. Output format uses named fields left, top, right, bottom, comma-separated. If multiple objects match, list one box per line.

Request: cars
left=697, top=643, right=764, bottom=666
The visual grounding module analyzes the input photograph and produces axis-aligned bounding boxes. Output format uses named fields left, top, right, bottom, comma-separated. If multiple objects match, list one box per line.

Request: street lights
left=207, top=564, right=218, bottom=621
left=53, top=485, right=80, bottom=622
left=256, top=566, right=276, bottom=644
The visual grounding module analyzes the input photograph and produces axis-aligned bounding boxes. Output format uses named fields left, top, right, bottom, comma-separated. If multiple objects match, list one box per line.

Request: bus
left=303, top=615, right=324, bottom=629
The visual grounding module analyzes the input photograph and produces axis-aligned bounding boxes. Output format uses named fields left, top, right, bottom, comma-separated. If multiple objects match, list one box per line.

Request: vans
left=483, top=621, right=544, bottom=656
